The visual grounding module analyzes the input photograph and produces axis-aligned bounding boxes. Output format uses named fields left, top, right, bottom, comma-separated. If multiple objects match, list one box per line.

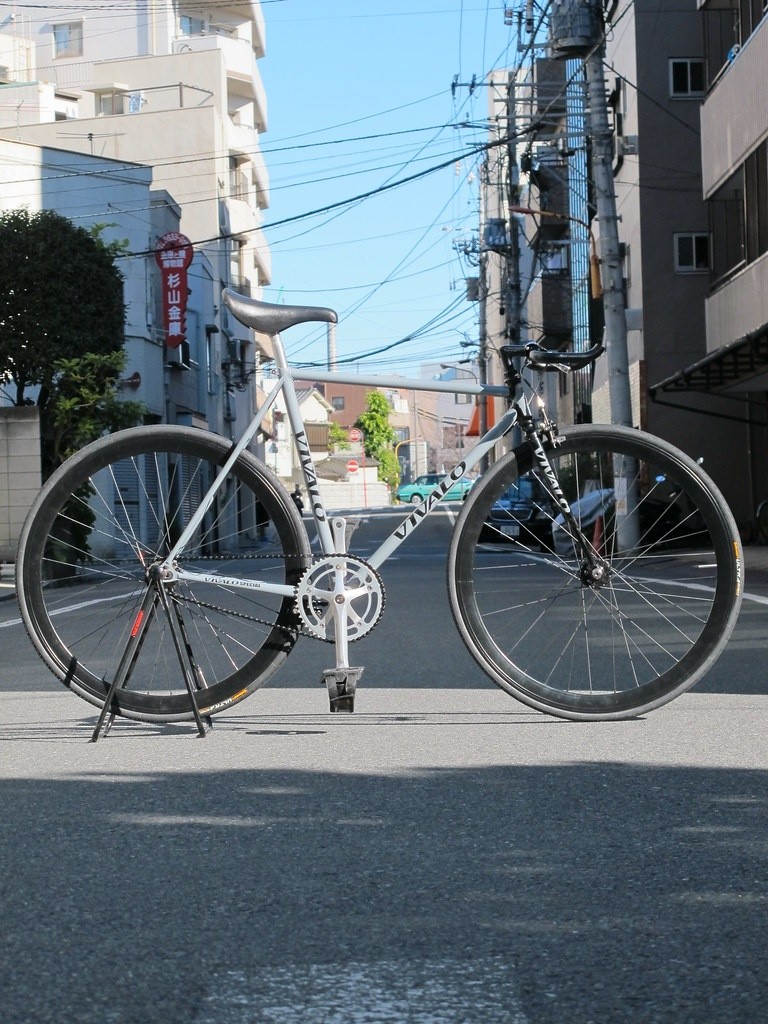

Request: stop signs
left=347, top=460, right=360, bottom=473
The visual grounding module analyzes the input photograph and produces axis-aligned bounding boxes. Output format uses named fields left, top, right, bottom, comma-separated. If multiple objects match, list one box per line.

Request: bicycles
left=753, top=498, right=768, bottom=545
left=12, top=291, right=747, bottom=752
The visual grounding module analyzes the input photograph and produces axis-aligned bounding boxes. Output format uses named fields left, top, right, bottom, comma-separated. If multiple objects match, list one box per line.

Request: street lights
left=502, top=207, right=641, bottom=558
left=439, top=361, right=487, bottom=475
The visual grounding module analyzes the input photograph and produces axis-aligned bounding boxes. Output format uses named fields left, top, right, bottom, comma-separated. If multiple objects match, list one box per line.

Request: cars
left=394, top=473, right=476, bottom=503
left=463, top=471, right=555, bottom=555
